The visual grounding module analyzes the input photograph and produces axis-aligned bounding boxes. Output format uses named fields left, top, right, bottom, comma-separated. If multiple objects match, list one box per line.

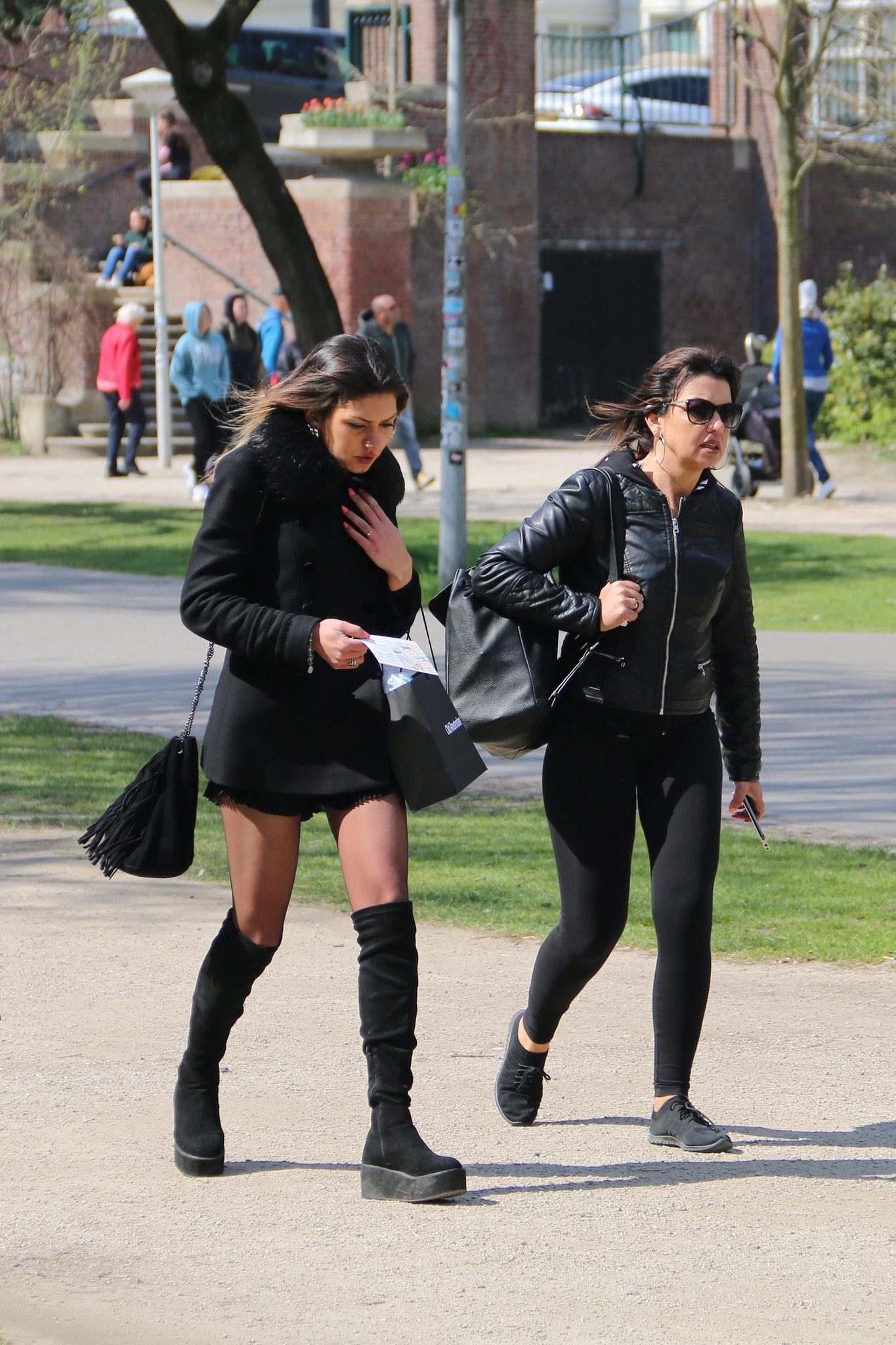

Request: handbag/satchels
left=78, top=735, right=199, bottom=879
left=369, top=608, right=488, bottom=814
left=427, top=466, right=619, bottom=759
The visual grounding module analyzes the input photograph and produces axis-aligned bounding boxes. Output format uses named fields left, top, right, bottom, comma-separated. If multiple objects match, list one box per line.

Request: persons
left=96, top=302, right=148, bottom=477
left=167, top=300, right=244, bottom=501
left=767, top=278, right=836, bottom=497
left=217, top=285, right=304, bottom=389
left=134, top=108, right=191, bottom=205
left=352, top=294, right=434, bottom=490
left=94, top=204, right=153, bottom=291
left=173, top=334, right=467, bottom=1202
left=469, top=348, right=765, bottom=1151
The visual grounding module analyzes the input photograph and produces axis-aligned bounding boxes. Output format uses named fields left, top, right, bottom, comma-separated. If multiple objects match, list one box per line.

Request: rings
left=349, top=658, right=357, bottom=667
left=631, top=597, right=639, bottom=611
left=367, top=528, right=373, bottom=536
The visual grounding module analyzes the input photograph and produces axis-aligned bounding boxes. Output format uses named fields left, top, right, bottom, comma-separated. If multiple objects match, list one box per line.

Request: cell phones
left=742, top=797, right=770, bottom=852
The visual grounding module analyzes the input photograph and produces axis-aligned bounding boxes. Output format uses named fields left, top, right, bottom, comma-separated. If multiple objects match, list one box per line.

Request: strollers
left=727, top=360, right=783, bottom=499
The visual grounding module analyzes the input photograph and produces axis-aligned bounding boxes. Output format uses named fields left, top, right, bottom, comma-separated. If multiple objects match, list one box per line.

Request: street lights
left=122, top=66, right=186, bottom=481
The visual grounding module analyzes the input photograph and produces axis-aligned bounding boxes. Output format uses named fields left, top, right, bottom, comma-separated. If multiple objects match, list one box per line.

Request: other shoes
left=105, top=466, right=126, bottom=478
left=106, top=276, right=123, bottom=290
left=193, top=485, right=208, bottom=503
left=416, top=475, right=433, bottom=491
left=95, top=277, right=108, bottom=288
left=122, top=463, right=147, bottom=477
left=818, top=480, right=836, bottom=501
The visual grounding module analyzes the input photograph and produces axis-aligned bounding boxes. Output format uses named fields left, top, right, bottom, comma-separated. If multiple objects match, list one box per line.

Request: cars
left=534, top=64, right=712, bottom=136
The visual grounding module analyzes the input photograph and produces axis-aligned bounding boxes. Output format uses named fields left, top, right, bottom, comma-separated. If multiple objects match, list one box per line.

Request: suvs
left=227, top=33, right=363, bottom=143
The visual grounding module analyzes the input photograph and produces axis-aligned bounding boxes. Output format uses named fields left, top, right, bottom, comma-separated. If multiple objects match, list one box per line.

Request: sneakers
left=495, top=1009, right=550, bottom=1125
left=647, top=1094, right=733, bottom=1152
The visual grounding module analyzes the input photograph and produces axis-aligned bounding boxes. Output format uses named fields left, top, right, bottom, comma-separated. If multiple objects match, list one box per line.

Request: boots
left=173, top=907, right=282, bottom=1177
left=350, top=901, right=468, bottom=1201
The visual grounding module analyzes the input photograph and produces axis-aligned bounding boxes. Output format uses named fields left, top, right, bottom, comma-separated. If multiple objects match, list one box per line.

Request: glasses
left=660, top=397, right=745, bottom=430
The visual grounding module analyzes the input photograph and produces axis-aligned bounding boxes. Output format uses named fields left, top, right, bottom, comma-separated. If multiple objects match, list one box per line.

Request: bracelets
left=307, top=632, right=314, bottom=674
